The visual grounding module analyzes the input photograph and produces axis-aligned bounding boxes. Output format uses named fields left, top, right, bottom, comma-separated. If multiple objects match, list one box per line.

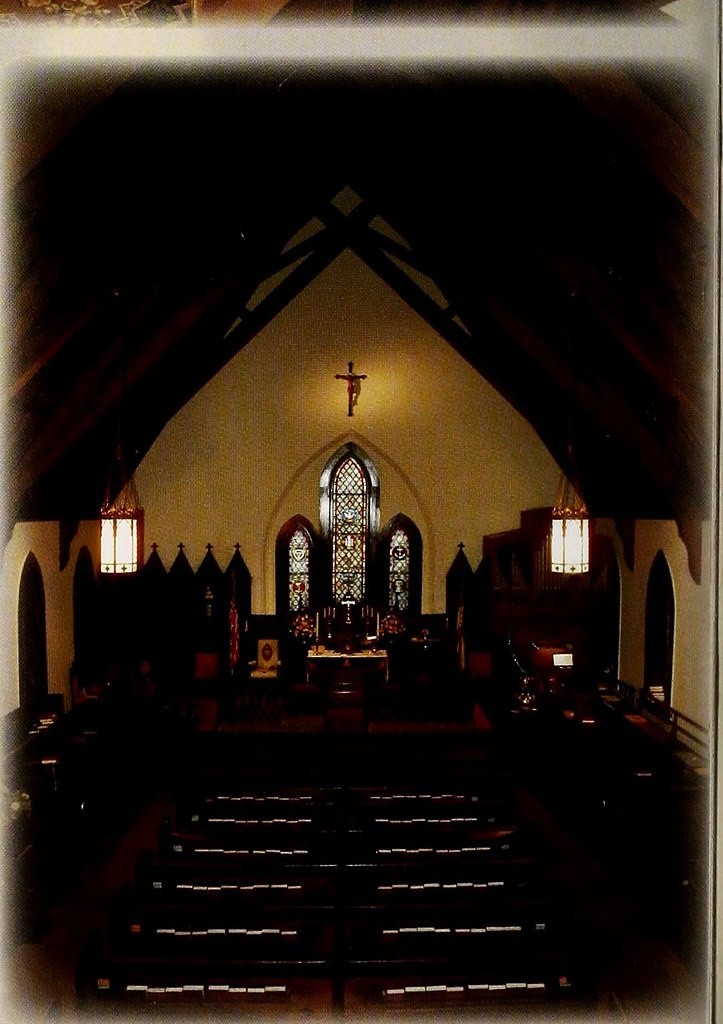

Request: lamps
left=98, top=445, right=141, bottom=574
left=550, top=461, right=592, bottom=574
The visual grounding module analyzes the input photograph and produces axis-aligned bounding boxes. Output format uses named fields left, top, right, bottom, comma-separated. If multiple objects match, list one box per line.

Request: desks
left=306, top=649, right=389, bottom=683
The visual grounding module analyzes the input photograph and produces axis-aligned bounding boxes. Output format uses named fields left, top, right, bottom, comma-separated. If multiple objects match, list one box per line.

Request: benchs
left=89, top=774, right=592, bottom=1002
left=597, top=674, right=636, bottom=715
left=624, top=688, right=678, bottom=747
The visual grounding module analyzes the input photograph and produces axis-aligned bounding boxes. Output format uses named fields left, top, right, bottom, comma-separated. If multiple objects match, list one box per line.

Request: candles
left=376, top=613, right=379, bottom=637
left=316, top=613, right=319, bottom=637
